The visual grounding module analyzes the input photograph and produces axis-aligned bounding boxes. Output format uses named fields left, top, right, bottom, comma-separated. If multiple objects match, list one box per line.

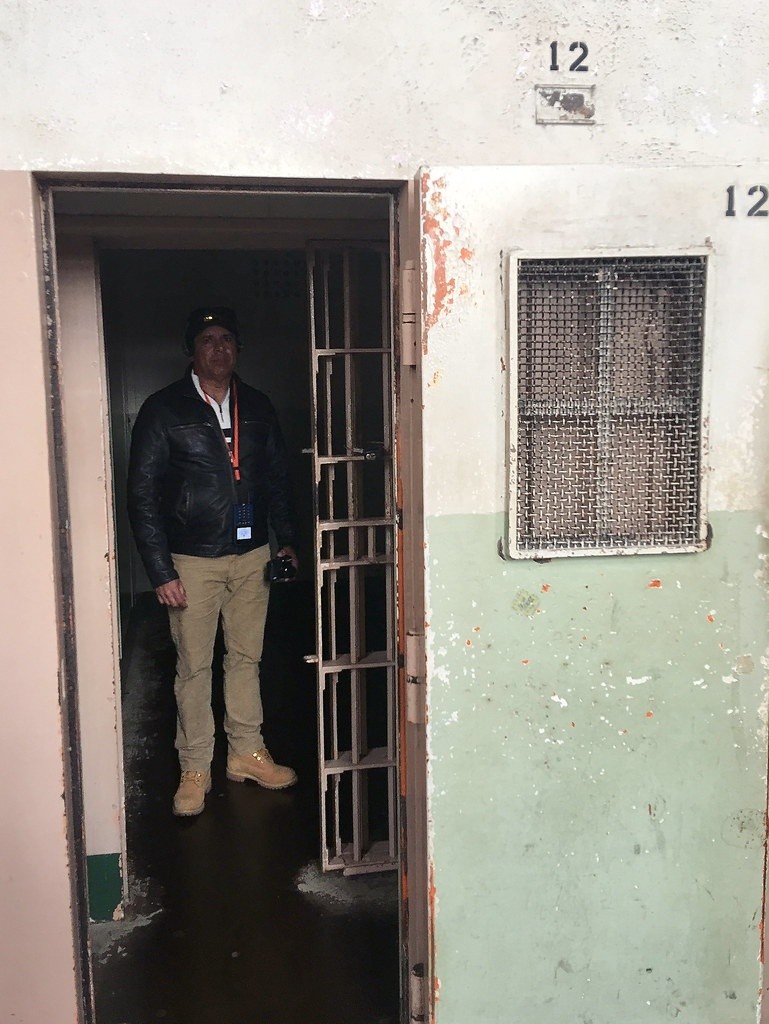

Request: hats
left=185, top=306, right=239, bottom=341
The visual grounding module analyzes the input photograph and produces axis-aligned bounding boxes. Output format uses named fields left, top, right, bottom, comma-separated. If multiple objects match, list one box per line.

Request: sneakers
left=172, top=767, right=212, bottom=816
left=227, top=749, right=297, bottom=789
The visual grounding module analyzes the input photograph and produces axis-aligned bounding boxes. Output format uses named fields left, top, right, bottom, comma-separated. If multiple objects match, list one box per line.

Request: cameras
left=267, top=555, right=296, bottom=584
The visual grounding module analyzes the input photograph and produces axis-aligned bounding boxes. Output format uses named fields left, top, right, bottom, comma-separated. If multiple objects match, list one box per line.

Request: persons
left=130, top=306, right=307, bottom=817
